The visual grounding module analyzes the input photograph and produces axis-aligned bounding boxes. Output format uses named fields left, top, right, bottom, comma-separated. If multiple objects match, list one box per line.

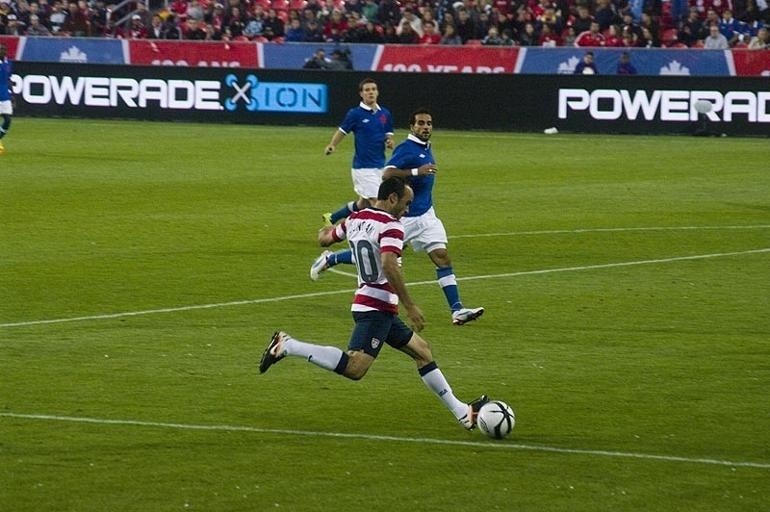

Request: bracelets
left=410, top=167, right=419, bottom=177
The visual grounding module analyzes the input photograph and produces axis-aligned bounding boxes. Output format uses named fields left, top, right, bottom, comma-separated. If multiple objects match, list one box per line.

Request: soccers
left=477, top=399, right=516, bottom=440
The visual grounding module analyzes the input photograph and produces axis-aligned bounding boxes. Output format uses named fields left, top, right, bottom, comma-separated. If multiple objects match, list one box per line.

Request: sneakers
left=322, top=213, right=332, bottom=226
left=459, top=395, right=488, bottom=433
left=311, top=250, right=333, bottom=281
left=451, top=307, right=484, bottom=325
left=260, top=331, right=291, bottom=374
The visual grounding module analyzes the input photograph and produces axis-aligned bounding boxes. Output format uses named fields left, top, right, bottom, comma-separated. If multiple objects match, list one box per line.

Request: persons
left=572, top=51, right=599, bottom=74
left=257, top=174, right=491, bottom=436
left=331, top=49, right=353, bottom=71
left=310, top=109, right=486, bottom=325
left=616, top=51, right=636, bottom=74
left=302, top=48, right=329, bottom=68
left=0, top=41, right=16, bottom=152
left=319, top=77, right=395, bottom=229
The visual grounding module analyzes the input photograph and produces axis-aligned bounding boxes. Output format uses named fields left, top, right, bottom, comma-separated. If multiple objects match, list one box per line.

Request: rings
left=428, top=169, right=433, bottom=172
